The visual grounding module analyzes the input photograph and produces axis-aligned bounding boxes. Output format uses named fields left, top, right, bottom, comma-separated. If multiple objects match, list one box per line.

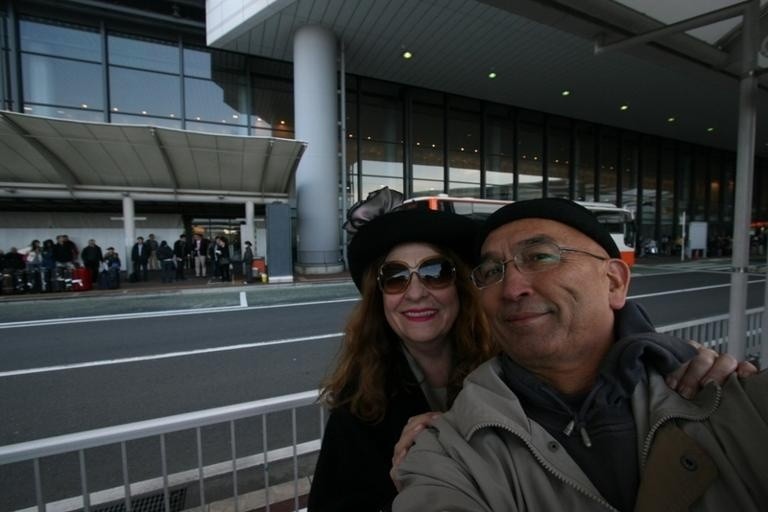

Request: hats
left=477, top=197, right=620, bottom=259
left=341, top=187, right=477, bottom=295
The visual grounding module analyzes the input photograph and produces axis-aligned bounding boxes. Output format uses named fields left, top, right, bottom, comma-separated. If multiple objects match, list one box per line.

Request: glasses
left=376, top=254, right=457, bottom=294
left=469, top=241, right=605, bottom=290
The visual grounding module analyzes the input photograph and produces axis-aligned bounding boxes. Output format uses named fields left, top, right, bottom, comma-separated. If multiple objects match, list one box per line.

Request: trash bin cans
left=251, top=256, right=265, bottom=282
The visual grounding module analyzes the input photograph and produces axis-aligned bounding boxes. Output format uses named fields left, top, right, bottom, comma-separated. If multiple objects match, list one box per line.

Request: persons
left=389, top=195, right=768, bottom=512
left=306, top=203, right=761, bottom=512
left=2, top=223, right=268, bottom=297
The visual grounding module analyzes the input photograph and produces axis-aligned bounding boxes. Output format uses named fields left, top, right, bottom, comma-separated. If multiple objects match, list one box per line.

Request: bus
left=399, top=191, right=640, bottom=272
